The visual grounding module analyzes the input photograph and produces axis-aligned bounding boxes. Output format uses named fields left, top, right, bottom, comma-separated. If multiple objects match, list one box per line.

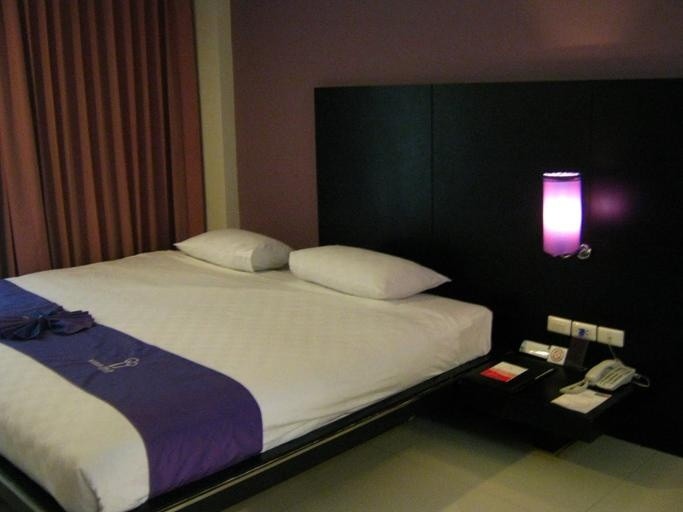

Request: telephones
left=585, top=358, right=636, bottom=391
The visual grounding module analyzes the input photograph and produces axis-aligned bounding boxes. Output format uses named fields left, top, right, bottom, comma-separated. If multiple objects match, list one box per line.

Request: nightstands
left=456, top=355, right=637, bottom=452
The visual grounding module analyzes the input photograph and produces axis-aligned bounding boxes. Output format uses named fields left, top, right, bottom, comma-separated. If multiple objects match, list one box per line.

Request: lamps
left=539, top=169, right=592, bottom=260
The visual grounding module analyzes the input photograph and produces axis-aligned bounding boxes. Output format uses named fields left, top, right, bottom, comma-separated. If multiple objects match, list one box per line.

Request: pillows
left=171, top=229, right=452, bottom=299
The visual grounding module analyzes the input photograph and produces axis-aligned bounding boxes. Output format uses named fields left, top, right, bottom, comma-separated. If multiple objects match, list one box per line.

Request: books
left=480, top=361, right=529, bottom=382
left=551, top=385, right=612, bottom=414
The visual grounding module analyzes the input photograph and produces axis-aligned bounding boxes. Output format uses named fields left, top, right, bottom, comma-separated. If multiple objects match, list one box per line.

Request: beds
left=1, top=249, right=512, bottom=512
